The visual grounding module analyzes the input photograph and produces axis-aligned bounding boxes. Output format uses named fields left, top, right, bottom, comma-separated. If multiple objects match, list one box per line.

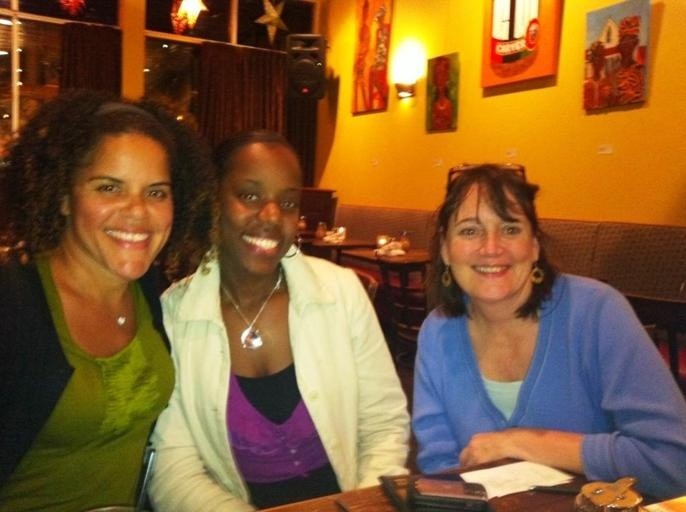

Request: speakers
left=285, top=34, right=325, bottom=99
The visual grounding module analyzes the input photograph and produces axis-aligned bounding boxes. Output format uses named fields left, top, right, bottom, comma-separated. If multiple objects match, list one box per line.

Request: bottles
left=575, top=480, right=643, bottom=512
left=432, top=58, right=452, bottom=129
left=295, top=215, right=411, bottom=252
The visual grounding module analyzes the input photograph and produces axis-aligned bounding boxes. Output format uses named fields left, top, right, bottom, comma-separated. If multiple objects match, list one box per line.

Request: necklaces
left=215, top=271, right=284, bottom=351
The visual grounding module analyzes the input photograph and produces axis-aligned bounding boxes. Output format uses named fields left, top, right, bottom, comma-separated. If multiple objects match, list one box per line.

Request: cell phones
left=410, top=477, right=488, bottom=512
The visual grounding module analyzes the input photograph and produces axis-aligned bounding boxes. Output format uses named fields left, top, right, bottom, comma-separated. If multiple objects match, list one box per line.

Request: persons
left=411, top=155, right=686, bottom=502
left=0, top=80, right=219, bottom=512
left=145, top=131, right=411, bottom=512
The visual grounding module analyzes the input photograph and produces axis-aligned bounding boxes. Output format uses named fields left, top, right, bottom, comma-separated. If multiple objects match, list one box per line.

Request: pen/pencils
left=530, top=485, right=582, bottom=495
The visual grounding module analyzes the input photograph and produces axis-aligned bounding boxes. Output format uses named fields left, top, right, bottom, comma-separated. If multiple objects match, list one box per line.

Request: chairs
left=625, top=293, right=686, bottom=396
left=373, top=252, right=429, bottom=361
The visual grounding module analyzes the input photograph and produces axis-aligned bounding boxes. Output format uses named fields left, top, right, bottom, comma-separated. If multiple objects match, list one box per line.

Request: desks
left=253, top=457, right=647, bottom=512
left=297, top=231, right=431, bottom=267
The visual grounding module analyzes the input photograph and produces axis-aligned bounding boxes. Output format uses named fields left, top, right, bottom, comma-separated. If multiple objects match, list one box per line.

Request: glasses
left=447, top=162, right=527, bottom=189
